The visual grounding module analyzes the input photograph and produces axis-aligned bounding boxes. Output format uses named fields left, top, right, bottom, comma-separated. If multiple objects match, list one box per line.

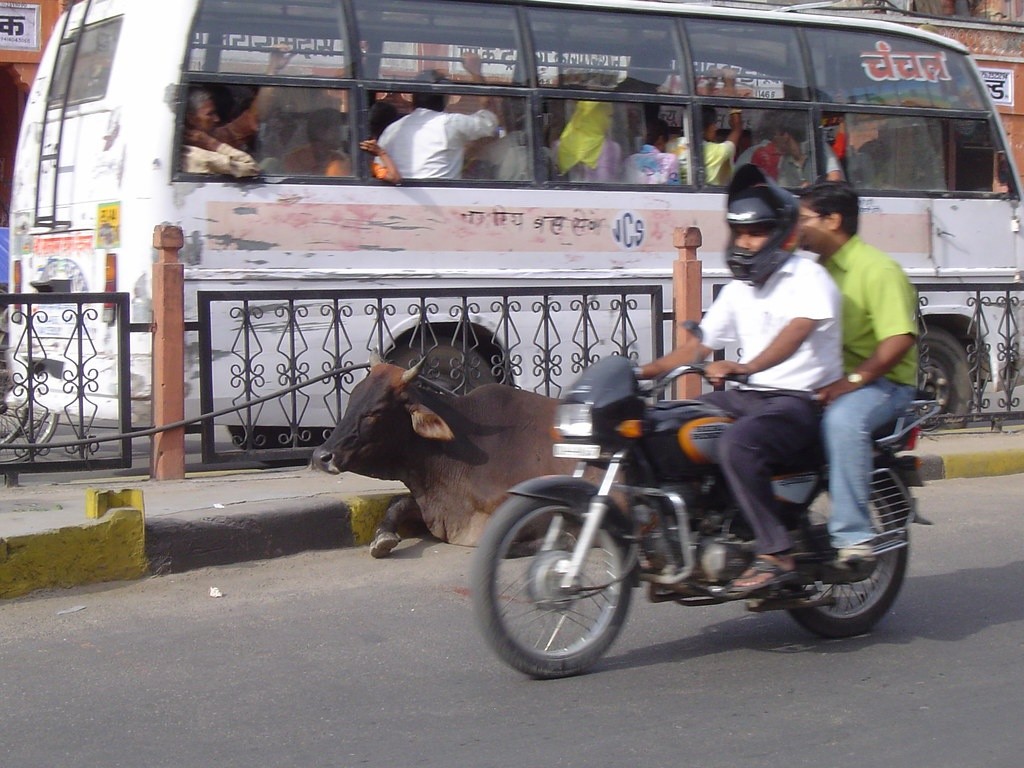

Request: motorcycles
left=469, top=356, right=945, bottom=683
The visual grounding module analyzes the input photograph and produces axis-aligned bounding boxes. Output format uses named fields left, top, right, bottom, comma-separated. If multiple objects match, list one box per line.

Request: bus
left=10, top=1, right=1024, bottom=453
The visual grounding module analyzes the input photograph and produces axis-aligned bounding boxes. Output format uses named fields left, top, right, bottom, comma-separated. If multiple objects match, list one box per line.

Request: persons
left=180, top=40, right=895, bottom=190
left=797, top=180, right=921, bottom=564
left=632, top=163, right=844, bottom=600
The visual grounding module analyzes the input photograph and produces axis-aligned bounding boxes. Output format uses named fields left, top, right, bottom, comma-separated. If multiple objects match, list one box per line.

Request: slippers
left=728, top=557, right=804, bottom=592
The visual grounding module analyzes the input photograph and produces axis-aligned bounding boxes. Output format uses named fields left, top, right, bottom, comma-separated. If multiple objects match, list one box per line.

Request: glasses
left=798, top=213, right=820, bottom=223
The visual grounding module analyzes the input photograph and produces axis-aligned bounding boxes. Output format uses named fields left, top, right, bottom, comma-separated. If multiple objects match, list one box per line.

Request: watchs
left=846, top=371, right=866, bottom=389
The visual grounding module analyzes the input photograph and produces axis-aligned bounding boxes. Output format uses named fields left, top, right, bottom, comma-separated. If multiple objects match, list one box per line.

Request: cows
left=312, top=348, right=658, bottom=559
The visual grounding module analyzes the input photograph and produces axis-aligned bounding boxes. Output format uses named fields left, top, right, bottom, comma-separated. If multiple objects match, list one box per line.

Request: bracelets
left=728, top=109, right=742, bottom=114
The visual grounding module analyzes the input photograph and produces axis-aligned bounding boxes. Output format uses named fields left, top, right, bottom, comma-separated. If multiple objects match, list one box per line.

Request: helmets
left=725, top=188, right=798, bottom=284
